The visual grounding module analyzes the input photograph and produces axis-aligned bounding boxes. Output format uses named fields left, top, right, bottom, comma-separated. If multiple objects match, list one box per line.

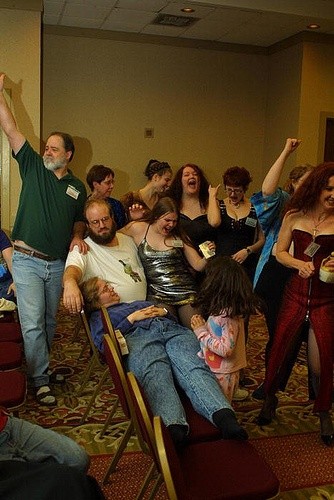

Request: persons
left=0, top=407, right=91, bottom=473
left=190, top=256, right=254, bottom=407
left=0, top=228, right=16, bottom=301
left=71, top=165, right=128, bottom=239
left=63, top=194, right=147, bottom=315
left=220, top=167, right=266, bottom=401
left=253, top=161, right=334, bottom=444
left=249, top=137, right=315, bottom=400
left=161, top=164, right=221, bottom=255
left=79, top=276, right=249, bottom=458
left=0, top=72, right=88, bottom=405
left=116, top=197, right=216, bottom=331
left=120, top=160, right=173, bottom=219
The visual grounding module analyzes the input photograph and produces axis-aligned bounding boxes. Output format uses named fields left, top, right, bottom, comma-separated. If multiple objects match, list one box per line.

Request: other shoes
left=252, top=383, right=266, bottom=399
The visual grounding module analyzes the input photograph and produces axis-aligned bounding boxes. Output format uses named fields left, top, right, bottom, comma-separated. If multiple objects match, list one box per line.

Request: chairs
left=0, top=309, right=28, bottom=419
left=78, top=307, right=280, bottom=500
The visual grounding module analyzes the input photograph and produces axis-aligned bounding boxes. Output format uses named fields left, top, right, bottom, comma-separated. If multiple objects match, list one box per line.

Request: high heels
left=320, top=414, right=334, bottom=446
left=256, top=396, right=278, bottom=425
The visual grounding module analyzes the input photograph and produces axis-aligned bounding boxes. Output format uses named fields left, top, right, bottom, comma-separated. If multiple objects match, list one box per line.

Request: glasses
left=98, top=281, right=109, bottom=296
left=225, top=188, right=244, bottom=194
left=88, top=215, right=112, bottom=226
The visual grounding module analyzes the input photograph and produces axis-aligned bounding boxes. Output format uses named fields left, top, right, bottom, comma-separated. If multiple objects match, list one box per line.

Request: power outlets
left=144, top=129, right=154, bottom=138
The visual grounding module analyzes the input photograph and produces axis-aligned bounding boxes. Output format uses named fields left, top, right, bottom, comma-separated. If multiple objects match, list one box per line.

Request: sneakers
left=232, top=390, right=250, bottom=401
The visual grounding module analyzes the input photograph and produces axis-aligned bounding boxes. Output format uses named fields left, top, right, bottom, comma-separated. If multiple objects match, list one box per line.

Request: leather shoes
left=222, top=417, right=248, bottom=440
left=170, top=430, right=193, bottom=448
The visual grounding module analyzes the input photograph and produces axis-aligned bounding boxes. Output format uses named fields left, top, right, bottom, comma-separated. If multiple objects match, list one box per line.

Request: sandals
left=46, top=369, right=64, bottom=384
left=34, top=386, right=58, bottom=406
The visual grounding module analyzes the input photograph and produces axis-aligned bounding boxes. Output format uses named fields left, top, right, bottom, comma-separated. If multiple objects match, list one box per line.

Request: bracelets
left=164, top=307, right=169, bottom=316
left=245, top=247, right=252, bottom=255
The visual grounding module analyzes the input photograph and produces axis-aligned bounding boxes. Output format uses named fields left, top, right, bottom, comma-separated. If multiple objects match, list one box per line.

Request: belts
left=14, top=245, right=58, bottom=261
